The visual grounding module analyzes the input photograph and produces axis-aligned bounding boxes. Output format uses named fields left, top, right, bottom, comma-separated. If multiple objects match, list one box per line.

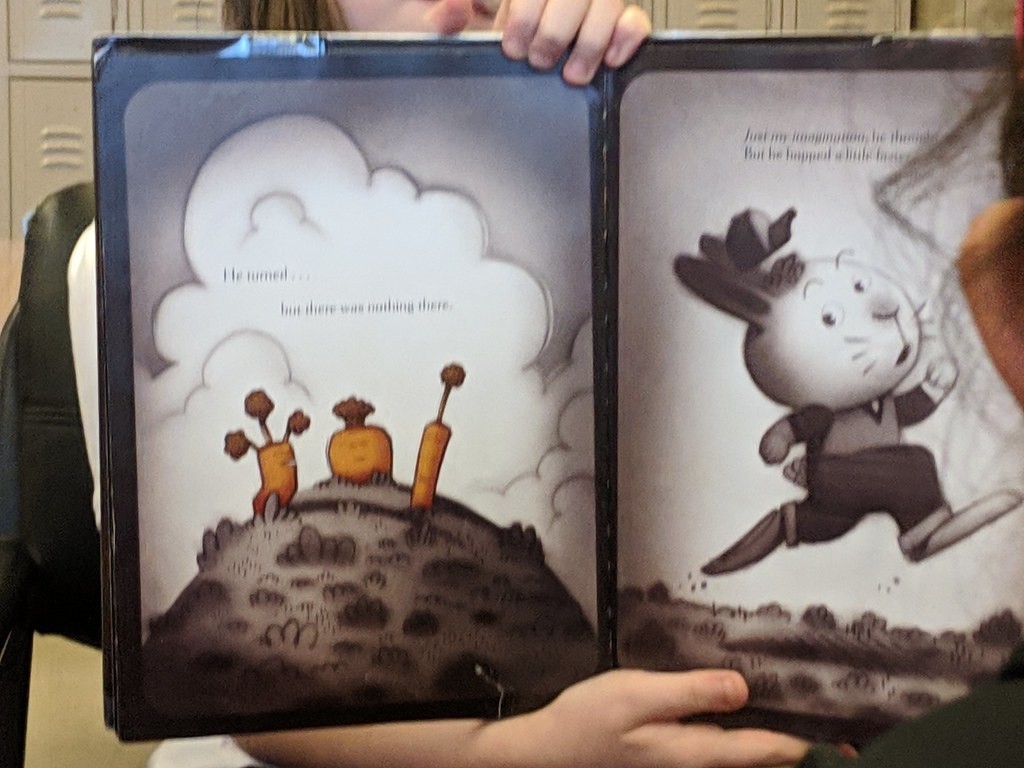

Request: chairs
left=0, top=182, right=95, bottom=768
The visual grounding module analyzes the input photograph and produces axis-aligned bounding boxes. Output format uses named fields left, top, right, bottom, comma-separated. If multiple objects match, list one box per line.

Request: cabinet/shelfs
left=0, top=0, right=912, bottom=322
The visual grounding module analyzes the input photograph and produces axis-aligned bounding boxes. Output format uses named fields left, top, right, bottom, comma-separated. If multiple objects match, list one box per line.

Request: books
left=86, top=32, right=1024, bottom=744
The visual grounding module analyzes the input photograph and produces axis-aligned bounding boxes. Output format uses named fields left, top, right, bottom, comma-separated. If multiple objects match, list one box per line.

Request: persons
left=0, top=0, right=858, bottom=768
left=799, top=38, right=1023, bottom=767
